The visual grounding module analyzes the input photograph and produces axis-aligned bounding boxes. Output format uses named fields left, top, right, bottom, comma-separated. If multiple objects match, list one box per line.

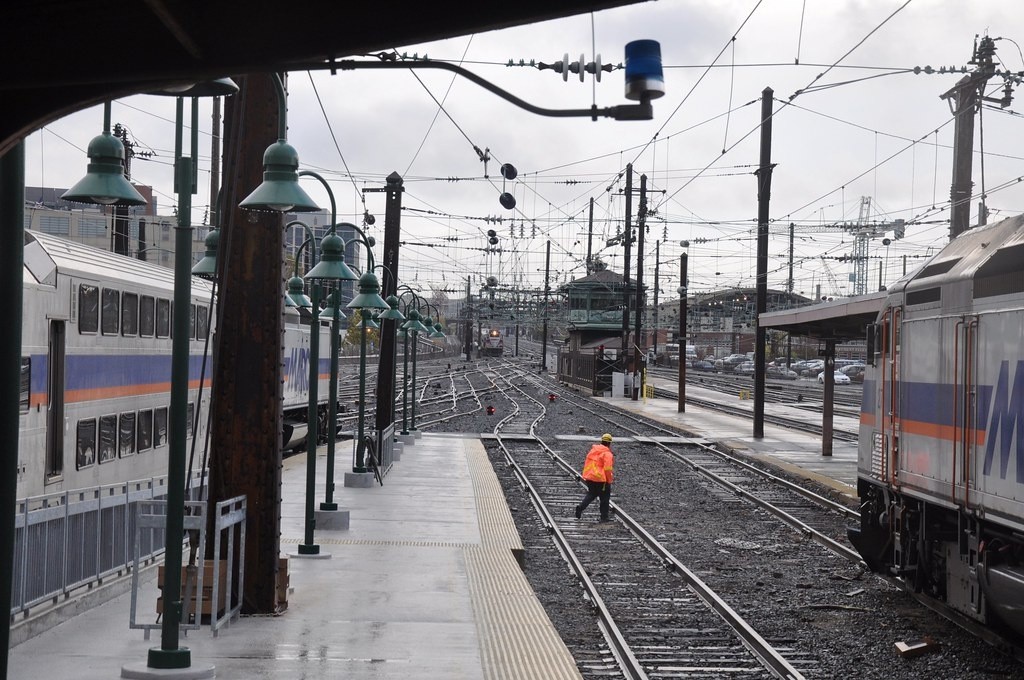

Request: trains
left=847, top=214, right=1023, bottom=654
left=473, top=328, right=503, bottom=358
left=17, top=227, right=342, bottom=518
left=665, top=344, right=696, bottom=355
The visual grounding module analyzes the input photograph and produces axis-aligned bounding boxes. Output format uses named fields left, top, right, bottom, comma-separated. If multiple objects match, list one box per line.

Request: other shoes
left=601, top=517, right=614, bottom=521
left=576, top=504, right=582, bottom=519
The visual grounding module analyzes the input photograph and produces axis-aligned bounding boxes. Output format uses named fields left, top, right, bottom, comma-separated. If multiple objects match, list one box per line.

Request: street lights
left=133, top=74, right=447, bottom=668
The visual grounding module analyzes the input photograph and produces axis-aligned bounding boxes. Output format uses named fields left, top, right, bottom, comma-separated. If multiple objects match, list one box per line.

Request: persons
left=576, top=433, right=614, bottom=522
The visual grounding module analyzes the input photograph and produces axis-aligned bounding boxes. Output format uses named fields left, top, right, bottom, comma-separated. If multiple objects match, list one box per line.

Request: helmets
left=602, top=433, right=613, bottom=442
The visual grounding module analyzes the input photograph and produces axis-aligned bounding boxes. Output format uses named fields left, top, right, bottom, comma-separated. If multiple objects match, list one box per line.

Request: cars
left=669, top=356, right=692, bottom=369
left=734, top=364, right=754, bottom=377
left=704, top=354, right=865, bottom=381
left=693, top=361, right=715, bottom=372
left=766, top=366, right=797, bottom=380
left=818, top=370, right=851, bottom=385
